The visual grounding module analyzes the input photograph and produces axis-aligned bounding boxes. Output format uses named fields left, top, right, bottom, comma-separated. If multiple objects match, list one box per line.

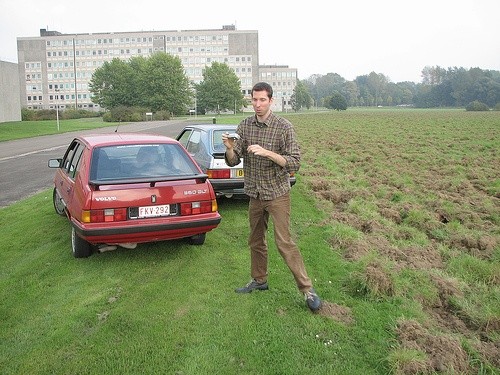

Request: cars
left=175, top=125, right=296, bottom=204
left=47, top=132, right=223, bottom=258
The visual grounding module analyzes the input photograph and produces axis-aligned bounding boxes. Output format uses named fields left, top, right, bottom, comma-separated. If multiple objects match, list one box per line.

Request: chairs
left=135, top=146, right=162, bottom=169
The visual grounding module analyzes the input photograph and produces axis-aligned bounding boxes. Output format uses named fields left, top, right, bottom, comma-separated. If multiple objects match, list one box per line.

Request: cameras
left=226, top=132, right=240, bottom=140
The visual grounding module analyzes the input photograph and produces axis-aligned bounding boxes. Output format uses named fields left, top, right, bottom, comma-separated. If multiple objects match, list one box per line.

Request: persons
left=221, top=82, right=323, bottom=312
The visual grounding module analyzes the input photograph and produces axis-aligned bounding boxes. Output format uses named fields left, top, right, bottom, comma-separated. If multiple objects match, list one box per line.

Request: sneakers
left=303, top=288, right=322, bottom=311
left=235, top=279, right=268, bottom=294
